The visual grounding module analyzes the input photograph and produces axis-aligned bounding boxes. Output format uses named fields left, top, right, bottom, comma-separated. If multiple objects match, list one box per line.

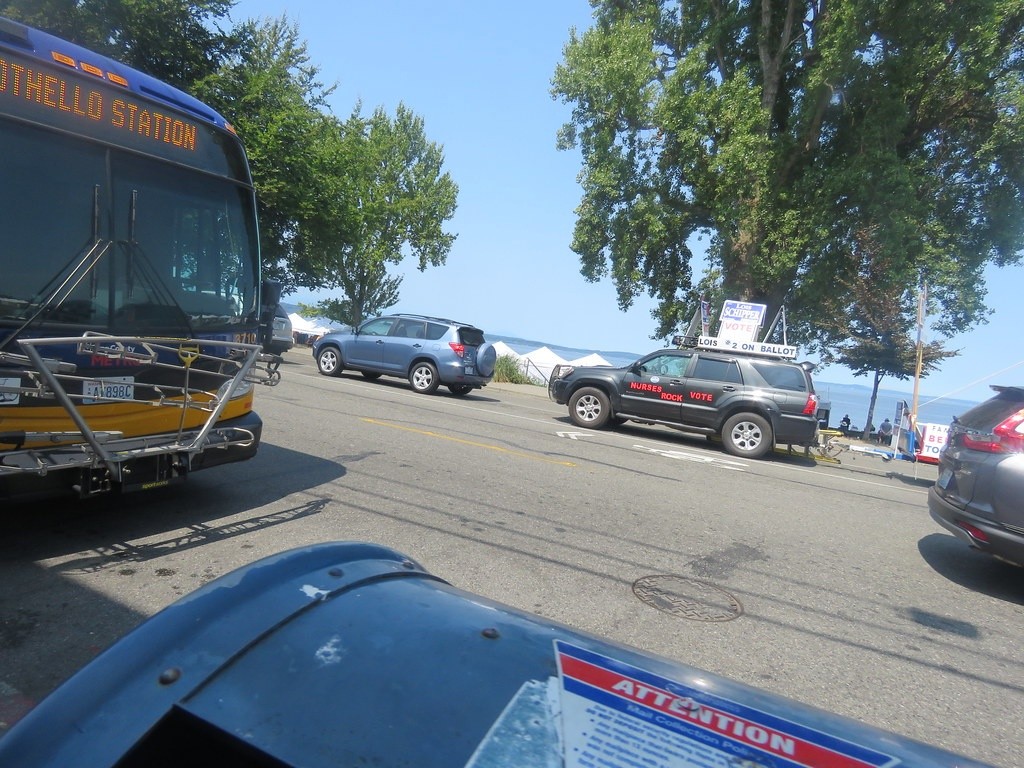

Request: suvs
left=312, top=314, right=496, bottom=394
left=548, top=335, right=818, bottom=459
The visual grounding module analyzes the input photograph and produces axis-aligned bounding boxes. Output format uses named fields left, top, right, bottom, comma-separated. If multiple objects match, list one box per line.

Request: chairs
left=397, top=326, right=431, bottom=339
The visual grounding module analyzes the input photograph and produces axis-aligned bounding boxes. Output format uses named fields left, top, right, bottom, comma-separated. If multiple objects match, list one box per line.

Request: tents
left=491, top=341, right=612, bottom=386
left=288, top=313, right=330, bottom=336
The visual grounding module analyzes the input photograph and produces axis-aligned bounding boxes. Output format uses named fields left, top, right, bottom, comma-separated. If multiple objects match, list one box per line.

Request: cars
left=928, top=385, right=1024, bottom=569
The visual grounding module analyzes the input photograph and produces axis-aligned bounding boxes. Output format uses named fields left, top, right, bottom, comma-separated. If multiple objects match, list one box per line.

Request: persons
left=878, top=418, right=892, bottom=436
left=843, top=415, right=850, bottom=430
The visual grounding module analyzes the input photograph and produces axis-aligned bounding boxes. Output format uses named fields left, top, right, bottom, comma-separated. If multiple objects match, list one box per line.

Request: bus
left=0, top=17, right=292, bottom=523
